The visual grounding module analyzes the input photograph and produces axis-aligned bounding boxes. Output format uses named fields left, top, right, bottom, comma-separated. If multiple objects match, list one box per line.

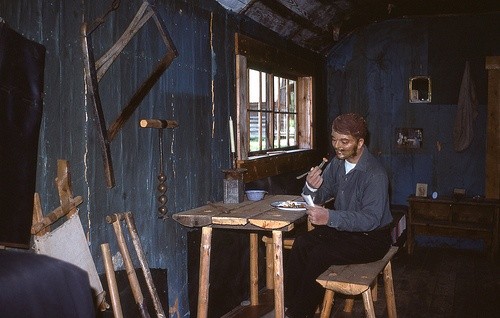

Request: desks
left=172, top=195, right=335, bottom=318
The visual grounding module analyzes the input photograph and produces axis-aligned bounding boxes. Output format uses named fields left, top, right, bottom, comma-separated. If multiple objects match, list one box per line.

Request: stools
left=316, top=246, right=398, bottom=318
left=261, top=236, right=295, bottom=289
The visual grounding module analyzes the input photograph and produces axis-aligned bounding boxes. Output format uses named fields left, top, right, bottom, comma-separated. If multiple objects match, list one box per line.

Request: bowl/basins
left=245, top=190, right=267, bottom=201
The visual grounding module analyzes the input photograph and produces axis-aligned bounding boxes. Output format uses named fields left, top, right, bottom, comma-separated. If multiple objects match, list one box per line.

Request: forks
left=296, top=160, right=326, bottom=180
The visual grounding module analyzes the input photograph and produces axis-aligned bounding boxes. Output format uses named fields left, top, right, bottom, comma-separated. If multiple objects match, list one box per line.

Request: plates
left=270, top=201, right=311, bottom=211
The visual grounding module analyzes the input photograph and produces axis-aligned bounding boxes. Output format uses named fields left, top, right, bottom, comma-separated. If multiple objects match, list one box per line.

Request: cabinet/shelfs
left=406, top=195, right=500, bottom=268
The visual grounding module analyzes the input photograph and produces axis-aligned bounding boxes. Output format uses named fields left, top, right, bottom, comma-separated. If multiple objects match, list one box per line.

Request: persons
left=263, top=113, right=392, bottom=318
